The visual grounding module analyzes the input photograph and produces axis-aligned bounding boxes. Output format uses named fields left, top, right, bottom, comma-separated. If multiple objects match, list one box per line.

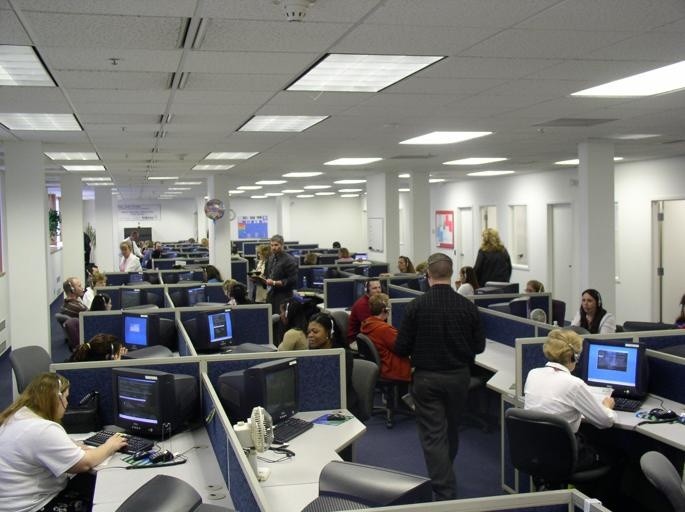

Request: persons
left=306, top=313, right=364, bottom=423
left=522, top=328, right=623, bottom=503
left=475, top=228, right=512, bottom=287
left=527, top=278, right=544, bottom=294
left=570, top=288, right=616, bottom=334
left=675, top=293, right=685, bottom=330
left=392, top=253, right=487, bottom=500
left=454, top=266, right=478, bottom=297
left=359, top=292, right=413, bottom=412
left=188, top=234, right=382, bottom=352
left=59, top=231, right=163, bottom=363
left=0, top=371, right=128, bottom=512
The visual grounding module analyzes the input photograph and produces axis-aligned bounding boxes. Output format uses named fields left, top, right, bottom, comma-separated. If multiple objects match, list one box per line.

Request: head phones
left=67, top=281, right=75, bottom=293
left=86, top=269, right=92, bottom=280
left=328, top=313, right=335, bottom=340
left=595, top=291, right=602, bottom=308
left=536, top=282, right=543, bottom=292
left=551, top=333, right=582, bottom=363
left=105, top=343, right=115, bottom=360
left=100, top=294, right=108, bottom=308
left=56, top=377, right=62, bottom=401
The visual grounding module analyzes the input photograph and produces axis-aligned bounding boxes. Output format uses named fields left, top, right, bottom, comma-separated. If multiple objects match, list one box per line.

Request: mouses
left=134, top=450, right=150, bottom=461
left=327, top=413, right=345, bottom=421
left=661, top=410, right=676, bottom=419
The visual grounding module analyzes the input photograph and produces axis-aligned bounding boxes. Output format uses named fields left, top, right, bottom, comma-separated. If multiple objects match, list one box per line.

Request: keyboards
left=265, top=417, right=313, bottom=444
left=612, top=396, right=641, bottom=413
left=84, top=429, right=154, bottom=455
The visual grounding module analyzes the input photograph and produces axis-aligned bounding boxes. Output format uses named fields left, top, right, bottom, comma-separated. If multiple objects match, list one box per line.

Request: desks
left=48, top=236, right=683, bottom=510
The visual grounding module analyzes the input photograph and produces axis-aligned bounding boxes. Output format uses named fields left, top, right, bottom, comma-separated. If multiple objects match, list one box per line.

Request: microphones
left=60, top=398, right=68, bottom=411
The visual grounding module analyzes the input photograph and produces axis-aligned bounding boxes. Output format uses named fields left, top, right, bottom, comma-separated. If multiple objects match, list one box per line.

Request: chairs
left=6, top=343, right=51, bottom=399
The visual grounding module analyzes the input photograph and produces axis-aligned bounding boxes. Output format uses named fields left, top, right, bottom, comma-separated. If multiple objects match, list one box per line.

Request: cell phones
left=148, top=449, right=173, bottom=462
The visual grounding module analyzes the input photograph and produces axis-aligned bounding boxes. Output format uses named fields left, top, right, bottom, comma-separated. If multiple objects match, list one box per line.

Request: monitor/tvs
left=121, top=312, right=176, bottom=349
left=300, top=460, right=432, bottom=512
left=120, top=241, right=231, bottom=307
left=120, top=344, right=174, bottom=360
left=183, top=308, right=236, bottom=353
left=227, top=342, right=277, bottom=352
left=233, top=238, right=474, bottom=331
left=475, top=281, right=519, bottom=294
left=114, top=475, right=235, bottom=512
left=582, top=339, right=650, bottom=398
left=215, top=358, right=299, bottom=430
left=488, top=296, right=531, bottom=319
left=113, top=368, right=198, bottom=442
